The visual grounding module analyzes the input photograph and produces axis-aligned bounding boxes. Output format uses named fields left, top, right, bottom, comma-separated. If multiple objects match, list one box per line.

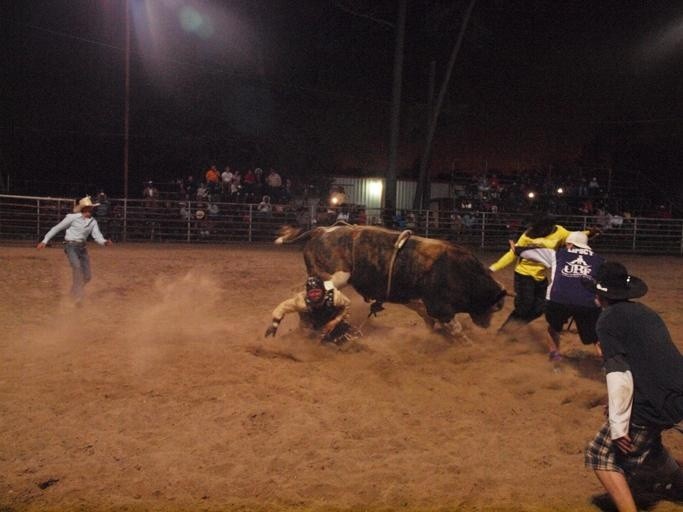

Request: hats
left=580, top=262, right=648, bottom=301
left=565, top=231, right=593, bottom=252
left=72, top=196, right=100, bottom=213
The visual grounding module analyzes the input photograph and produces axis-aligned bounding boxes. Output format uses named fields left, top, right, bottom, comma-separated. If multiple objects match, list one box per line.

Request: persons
left=579, top=263, right=682, bottom=511
left=35, top=194, right=111, bottom=299
left=264, top=273, right=354, bottom=344
left=82, top=157, right=683, bottom=252
left=483, top=204, right=589, bottom=332
left=507, top=228, right=602, bottom=366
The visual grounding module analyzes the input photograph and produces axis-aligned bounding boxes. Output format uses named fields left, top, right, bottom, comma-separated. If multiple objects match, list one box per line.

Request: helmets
left=306, top=274, right=323, bottom=291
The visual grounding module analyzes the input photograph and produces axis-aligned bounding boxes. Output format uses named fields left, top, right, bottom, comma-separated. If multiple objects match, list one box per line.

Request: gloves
left=265, top=322, right=278, bottom=337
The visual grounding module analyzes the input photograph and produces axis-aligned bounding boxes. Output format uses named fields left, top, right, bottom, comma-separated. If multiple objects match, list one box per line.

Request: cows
left=272, top=222, right=520, bottom=351
